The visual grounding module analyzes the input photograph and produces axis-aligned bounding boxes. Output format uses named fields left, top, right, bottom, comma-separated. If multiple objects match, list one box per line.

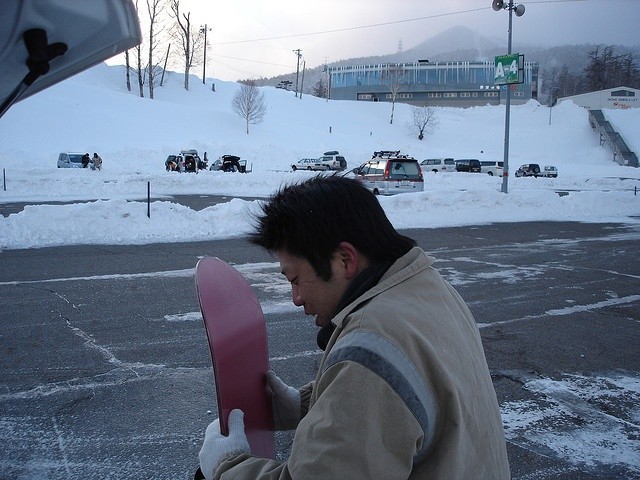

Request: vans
left=352, top=150, right=424, bottom=196
left=453, top=159, right=482, bottom=175
left=57, top=152, right=94, bottom=169
left=420, top=157, right=456, bottom=171
left=479, top=160, right=510, bottom=178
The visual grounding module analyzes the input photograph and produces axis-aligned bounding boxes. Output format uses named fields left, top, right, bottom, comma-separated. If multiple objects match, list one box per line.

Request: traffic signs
left=494, top=52, right=520, bottom=84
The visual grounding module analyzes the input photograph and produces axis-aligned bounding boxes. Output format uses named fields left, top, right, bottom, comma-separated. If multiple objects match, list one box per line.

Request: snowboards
left=194, top=255, right=277, bottom=460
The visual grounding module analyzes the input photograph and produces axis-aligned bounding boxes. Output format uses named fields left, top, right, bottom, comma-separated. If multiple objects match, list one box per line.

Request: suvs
left=209, top=154, right=238, bottom=173
left=318, top=150, right=348, bottom=172
left=514, top=163, right=541, bottom=178
left=165, top=148, right=209, bottom=175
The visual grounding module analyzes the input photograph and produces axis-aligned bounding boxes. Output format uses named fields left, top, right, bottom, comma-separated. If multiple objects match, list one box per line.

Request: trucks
left=541, top=166, right=559, bottom=179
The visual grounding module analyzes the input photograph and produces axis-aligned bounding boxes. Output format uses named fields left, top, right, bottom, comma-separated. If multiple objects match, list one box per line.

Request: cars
left=290, top=158, right=328, bottom=172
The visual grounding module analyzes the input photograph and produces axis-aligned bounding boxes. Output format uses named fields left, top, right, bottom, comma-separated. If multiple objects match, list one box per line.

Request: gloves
left=266, top=370, right=302, bottom=431
left=198, top=409, right=250, bottom=480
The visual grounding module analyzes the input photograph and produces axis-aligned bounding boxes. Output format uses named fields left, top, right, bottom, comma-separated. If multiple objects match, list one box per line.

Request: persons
left=91, top=153, right=102, bottom=170
left=82, top=154, right=92, bottom=167
left=198, top=175, right=515, bottom=479
